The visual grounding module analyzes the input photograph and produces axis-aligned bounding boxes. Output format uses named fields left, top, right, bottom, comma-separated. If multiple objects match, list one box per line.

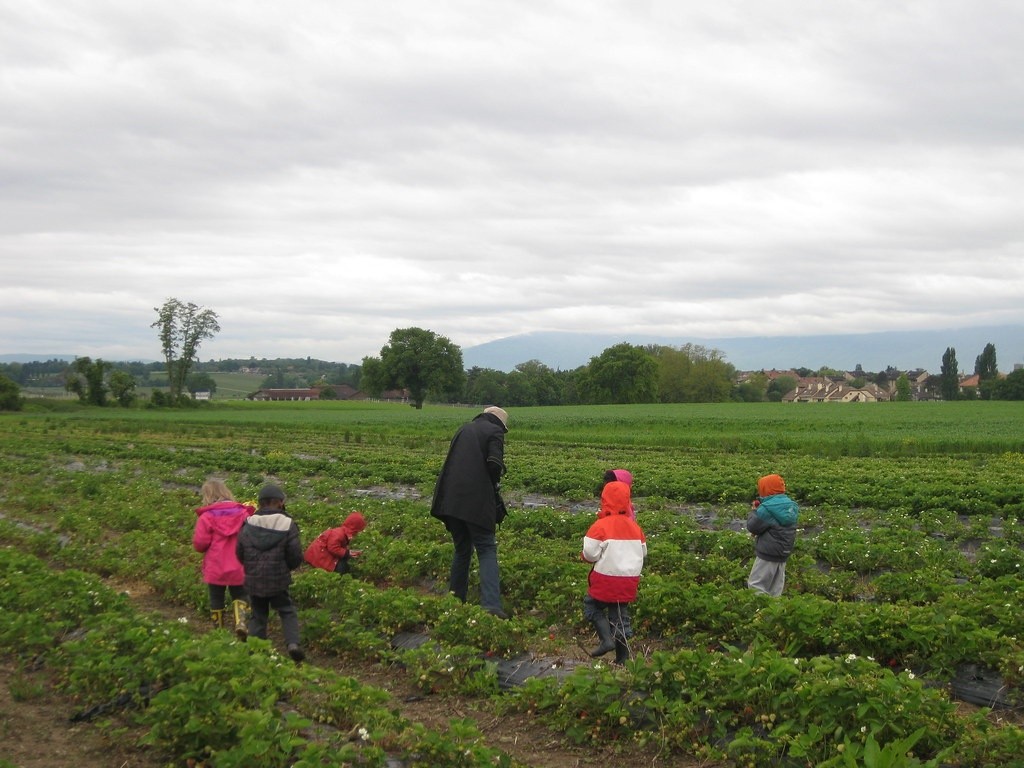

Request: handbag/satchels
left=487, top=457, right=509, bottom=524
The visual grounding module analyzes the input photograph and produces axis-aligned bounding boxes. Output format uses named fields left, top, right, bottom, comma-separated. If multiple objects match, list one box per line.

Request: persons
left=580, top=469, right=647, bottom=667
left=747, top=474, right=798, bottom=596
left=304, top=512, right=366, bottom=575
left=431, top=406, right=508, bottom=620
left=193, top=477, right=258, bottom=642
left=235, top=484, right=306, bottom=663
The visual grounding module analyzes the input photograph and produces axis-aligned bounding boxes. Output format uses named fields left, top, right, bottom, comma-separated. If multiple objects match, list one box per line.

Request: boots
left=613, top=634, right=627, bottom=666
left=210, top=609, right=224, bottom=632
left=232, top=598, right=248, bottom=642
left=590, top=618, right=616, bottom=656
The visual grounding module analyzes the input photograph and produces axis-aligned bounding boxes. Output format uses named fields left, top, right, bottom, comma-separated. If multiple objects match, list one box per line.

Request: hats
left=258, top=484, right=285, bottom=512
left=483, top=406, right=509, bottom=433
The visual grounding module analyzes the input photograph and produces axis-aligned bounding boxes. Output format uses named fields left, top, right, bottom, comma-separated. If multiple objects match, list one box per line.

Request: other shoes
left=287, top=642, right=305, bottom=662
left=485, top=608, right=511, bottom=621
